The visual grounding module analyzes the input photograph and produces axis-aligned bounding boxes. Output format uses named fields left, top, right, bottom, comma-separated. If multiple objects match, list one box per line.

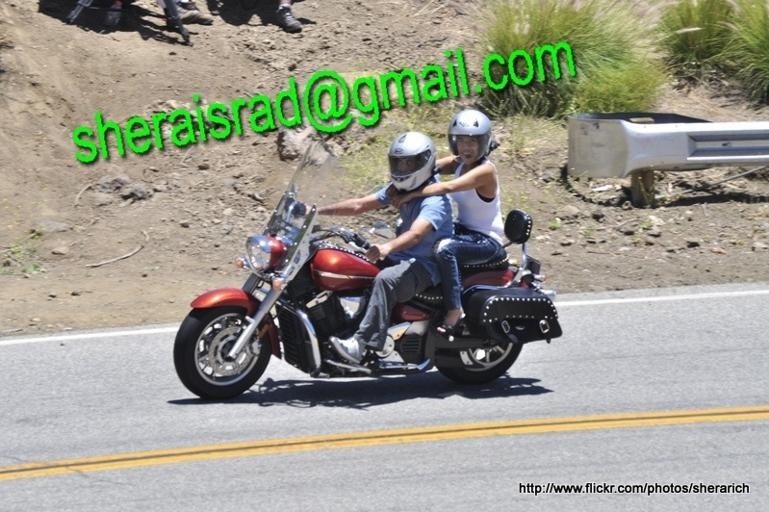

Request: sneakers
left=329, top=335, right=364, bottom=364
left=274, top=6, right=302, bottom=32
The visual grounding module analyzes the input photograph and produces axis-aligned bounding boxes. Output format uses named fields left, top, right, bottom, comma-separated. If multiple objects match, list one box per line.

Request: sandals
left=437, top=308, right=467, bottom=335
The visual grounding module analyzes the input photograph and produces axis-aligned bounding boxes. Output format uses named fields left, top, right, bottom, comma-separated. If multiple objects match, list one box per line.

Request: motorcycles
left=174, top=139, right=563, bottom=400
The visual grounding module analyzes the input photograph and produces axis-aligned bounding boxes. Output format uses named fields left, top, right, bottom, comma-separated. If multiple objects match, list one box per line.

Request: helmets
left=387, top=132, right=437, bottom=191
left=448, top=109, right=492, bottom=165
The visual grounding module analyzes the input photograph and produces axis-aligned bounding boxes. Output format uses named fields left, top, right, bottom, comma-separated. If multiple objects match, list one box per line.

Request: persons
left=389, top=109, right=510, bottom=328
left=37, top=0, right=304, bottom=33
left=295, top=130, right=454, bottom=364
